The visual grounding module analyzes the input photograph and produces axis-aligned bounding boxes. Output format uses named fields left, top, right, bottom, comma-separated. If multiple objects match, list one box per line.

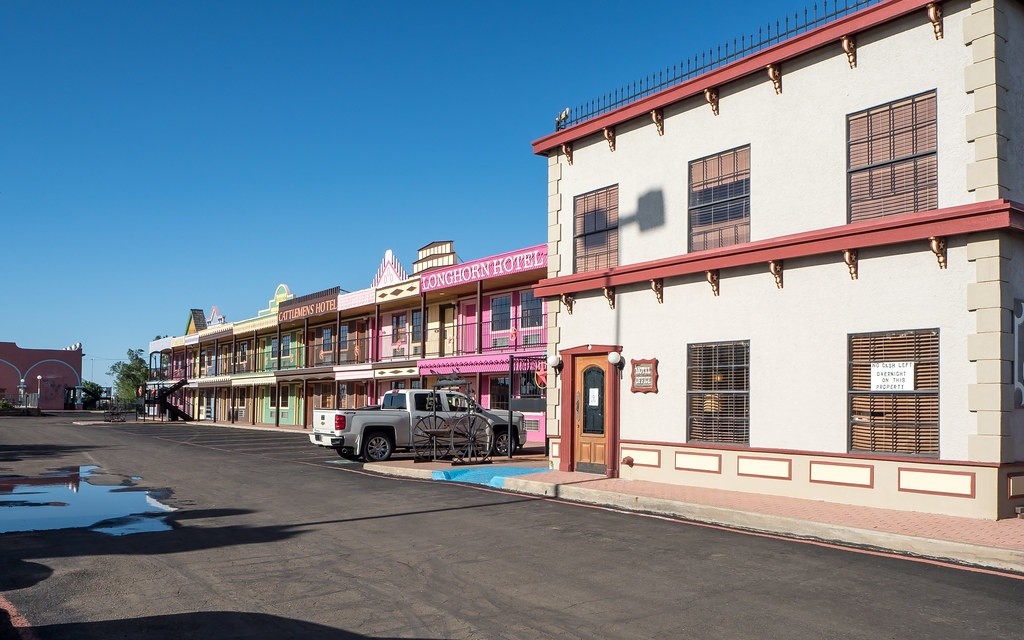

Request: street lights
left=37, top=375, right=43, bottom=416
left=21, top=379, right=25, bottom=406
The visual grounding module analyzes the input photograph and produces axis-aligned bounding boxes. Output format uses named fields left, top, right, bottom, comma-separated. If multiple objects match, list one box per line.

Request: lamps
left=548, top=355, right=564, bottom=373
left=608, top=351, right=625, bottom=371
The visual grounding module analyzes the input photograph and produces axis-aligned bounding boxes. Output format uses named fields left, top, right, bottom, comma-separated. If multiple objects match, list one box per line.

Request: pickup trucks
left=308, top=387, right=528, bottom=463
